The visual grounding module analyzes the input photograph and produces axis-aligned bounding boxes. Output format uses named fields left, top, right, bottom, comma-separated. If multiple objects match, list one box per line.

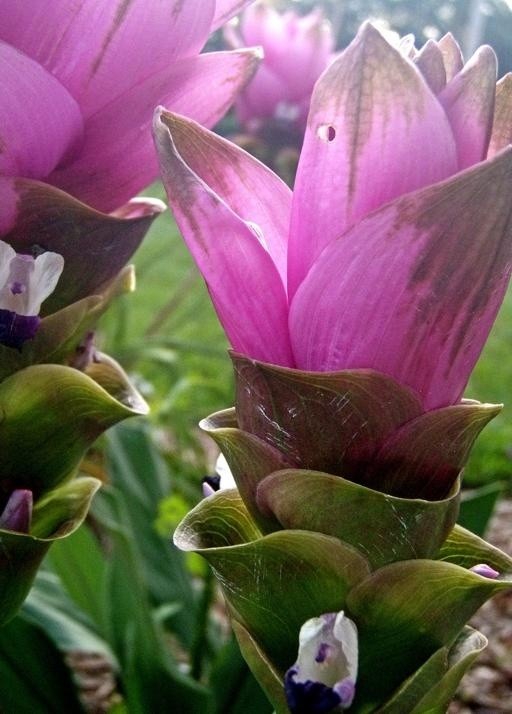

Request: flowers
left=0, top=0, right=510, bottom=713
